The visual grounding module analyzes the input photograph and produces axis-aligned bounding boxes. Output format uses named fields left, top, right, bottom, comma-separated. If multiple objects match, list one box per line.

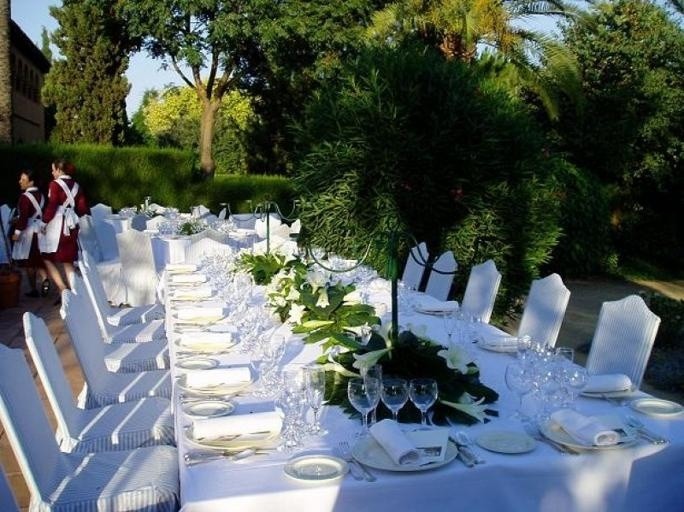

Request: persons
left=10, top=168, right=51, bottom=298
left=37, top=157, right=91, bottom=305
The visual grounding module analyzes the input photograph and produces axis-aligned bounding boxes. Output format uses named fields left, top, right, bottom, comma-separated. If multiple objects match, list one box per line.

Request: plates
left=281, top=453, right=350, bottom=484
left=181, top=400, right=235, bottom=417
left=180, top=421, right=283, bottom=452
left=476, top=433, right=538, bottom=454
left=176, top=357, right=219, bottom=370
left=353, top=435, right=459, bottom=474
left=540, top=387, right=684, bottom=456
left=167, top=263, right=237, bottom=354
left=176, top=374, right=256, bottom=395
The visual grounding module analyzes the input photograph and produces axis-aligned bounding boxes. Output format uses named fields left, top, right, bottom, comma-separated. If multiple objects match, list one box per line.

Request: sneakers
left=41, top=279, right=50, bottom=297
left=53, top=295, right=61, bottom=305
left=24, top=289, right=40, bottom=298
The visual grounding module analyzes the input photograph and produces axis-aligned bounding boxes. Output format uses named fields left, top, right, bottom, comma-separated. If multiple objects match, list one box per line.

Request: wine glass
left=444, top=306, right=588, bottom=443
left=309, top=249, right=351, bottom=282
left=352, top=264, right=418, bottom=316
left=347, top=363, right=438, bottom=439
left=199, top=250, right=325, bottom=455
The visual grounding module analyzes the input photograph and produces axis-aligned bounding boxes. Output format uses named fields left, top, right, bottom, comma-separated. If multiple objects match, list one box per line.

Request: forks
left=337, top=440, right=375, bottom=483
